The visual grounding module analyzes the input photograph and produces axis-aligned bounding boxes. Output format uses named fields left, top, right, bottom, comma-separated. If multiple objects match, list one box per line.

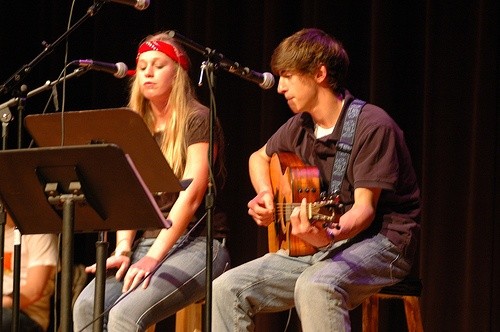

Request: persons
left=2, top=208, right=60, bottom=332
left=211, top=29, right=420, bottom=332
left=73, top=33, right=231, bottom=332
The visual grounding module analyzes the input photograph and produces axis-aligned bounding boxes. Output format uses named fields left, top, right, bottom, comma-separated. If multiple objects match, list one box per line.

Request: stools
left=361, top=276, right=424, bottom=332
left=145, top=296, right=206, bottom=332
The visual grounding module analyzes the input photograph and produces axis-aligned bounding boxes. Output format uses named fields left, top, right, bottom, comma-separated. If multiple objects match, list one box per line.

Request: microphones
left=112, top=0, right=150, bottom=11
left=73, top=60, right=128, bottom=78
left=218, top=61, right=275, bottom=89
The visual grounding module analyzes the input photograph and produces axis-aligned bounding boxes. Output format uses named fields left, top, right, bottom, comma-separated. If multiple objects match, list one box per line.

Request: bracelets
left=317, top=227, right=335, bottom=253
left=111, top=250, right=132, bottom=257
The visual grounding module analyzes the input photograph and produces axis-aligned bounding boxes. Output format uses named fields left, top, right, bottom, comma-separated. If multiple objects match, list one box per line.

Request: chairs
left=70, top=265, right=89, bottom=308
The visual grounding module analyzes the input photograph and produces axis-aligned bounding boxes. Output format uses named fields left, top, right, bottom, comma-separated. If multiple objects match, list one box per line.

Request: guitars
left=267, top=152, right=346, bottom=257
